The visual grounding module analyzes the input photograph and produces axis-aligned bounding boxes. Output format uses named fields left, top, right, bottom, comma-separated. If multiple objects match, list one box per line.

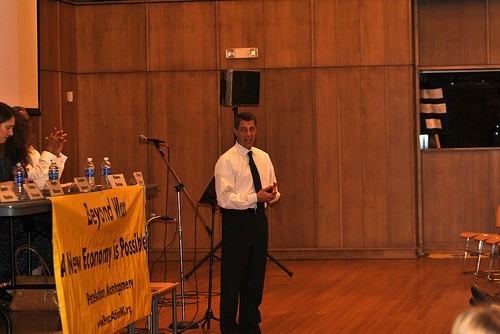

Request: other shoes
left=470, top=285, right=493, bottom=304
left=469, top=297, right=480, bottom=308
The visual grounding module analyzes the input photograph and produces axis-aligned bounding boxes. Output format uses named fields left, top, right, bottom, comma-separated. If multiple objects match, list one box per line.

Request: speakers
left=220, top=70, right=261, bottom=107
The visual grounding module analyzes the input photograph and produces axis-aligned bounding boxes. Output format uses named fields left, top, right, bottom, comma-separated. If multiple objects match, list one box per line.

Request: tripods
left=179, top=180, right=221, bottom=333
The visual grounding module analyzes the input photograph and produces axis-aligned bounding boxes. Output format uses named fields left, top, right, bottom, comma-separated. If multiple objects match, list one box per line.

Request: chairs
left=460, top=205, right=500, bottom=305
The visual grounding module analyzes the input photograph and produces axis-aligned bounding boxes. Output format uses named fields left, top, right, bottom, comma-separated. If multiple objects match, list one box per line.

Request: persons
left=13, top=106, right=68, bottom=187
left=215, top=112, right=281, bottom=334
left=469, top=285, right=500, bottom=306
left=0, top=102, right=30, bottom=182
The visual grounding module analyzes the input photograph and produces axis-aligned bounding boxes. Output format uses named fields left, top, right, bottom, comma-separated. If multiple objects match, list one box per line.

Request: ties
left=247, top=151, right=265, bottom=215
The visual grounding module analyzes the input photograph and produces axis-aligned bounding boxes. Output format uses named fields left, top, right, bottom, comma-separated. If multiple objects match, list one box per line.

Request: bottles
left=101, top=157, right=112, bottom=189
left=85, top=158, right=95, bottom=191
left=49, top=161, right=59, bottom=185
left=12, top=163, right=27, bottom=201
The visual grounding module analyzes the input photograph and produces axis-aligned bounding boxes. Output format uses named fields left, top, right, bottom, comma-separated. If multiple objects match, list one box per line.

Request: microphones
left=151, top=213, right=176, bottom=221
left=143, top=137, right=167, bottom=145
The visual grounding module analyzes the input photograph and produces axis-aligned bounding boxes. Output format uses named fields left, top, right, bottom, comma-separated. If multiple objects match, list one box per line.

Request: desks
left=0, top=183, right=160, bottom=334
left=128, top=282, right=179, bottom=334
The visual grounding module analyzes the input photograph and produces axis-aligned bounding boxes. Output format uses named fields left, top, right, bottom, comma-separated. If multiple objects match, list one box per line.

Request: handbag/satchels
left=8, top=244, right=60, bottom=311
